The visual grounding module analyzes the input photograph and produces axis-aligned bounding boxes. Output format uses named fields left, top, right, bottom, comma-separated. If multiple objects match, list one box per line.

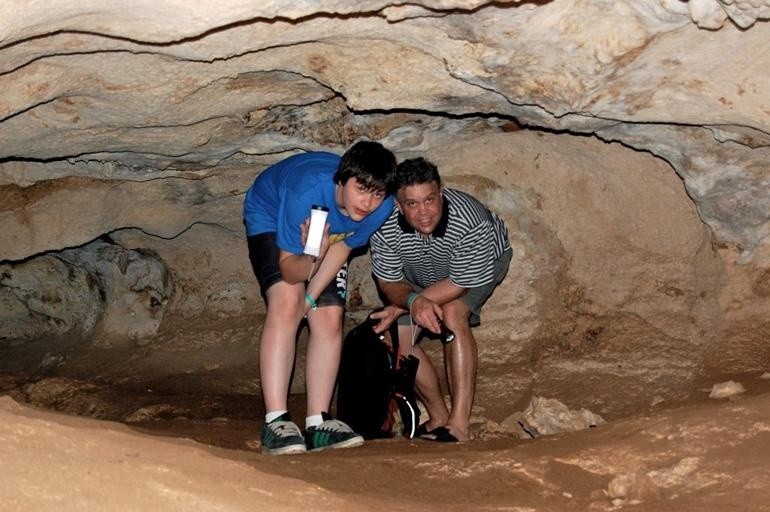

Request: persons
left=243, top=142, right=398, bottom=456
left=370, top=156, right=513, bottom=442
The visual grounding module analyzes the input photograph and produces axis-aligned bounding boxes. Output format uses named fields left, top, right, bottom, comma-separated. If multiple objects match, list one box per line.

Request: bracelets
left=304, top=293, right=317, bottom=310
left=405, top=293, right=421, bottom=309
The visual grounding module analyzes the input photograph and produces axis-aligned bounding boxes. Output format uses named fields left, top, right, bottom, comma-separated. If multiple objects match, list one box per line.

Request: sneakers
left=260, top=411, right=365, bottom=457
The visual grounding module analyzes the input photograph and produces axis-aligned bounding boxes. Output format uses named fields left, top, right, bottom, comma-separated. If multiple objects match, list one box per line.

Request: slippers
left=410, top=420, right=472, bottom=443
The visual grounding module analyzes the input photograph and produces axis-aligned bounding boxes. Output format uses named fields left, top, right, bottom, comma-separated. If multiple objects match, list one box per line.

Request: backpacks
left=336, top=306, right=401, bottom=441
